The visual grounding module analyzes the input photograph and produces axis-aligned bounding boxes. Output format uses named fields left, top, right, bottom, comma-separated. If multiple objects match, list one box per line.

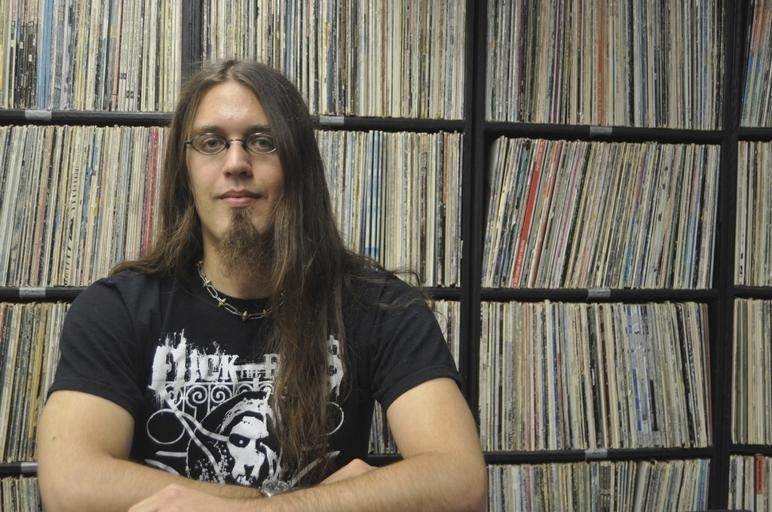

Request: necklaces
left=192, top=258, right=287, bottom=321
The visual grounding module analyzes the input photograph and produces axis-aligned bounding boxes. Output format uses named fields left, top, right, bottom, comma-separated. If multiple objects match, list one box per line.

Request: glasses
left=183, top=129, right=278, bottom=155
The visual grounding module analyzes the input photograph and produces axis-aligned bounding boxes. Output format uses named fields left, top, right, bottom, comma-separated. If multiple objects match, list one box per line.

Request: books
left=81, top=125, right=110, bottom=284
left=385, top=130, right=420, bottom=286
left=396, top=1, right=420, bottom=119
left=732, top=298, right=771, bottom=445
left=350, top=130, right=388, bottom=270
left=479, top=301, right=714, bottom=451
left=424, top=300, right=460, bottom=373
left=0, top=474, right=43, bottom=512
left=441, top=130, right=464, bottom=286
left=288, top=1, right=327, bottom=115
left=482, top=135, right=721, bottom=289
left=370, top=0, right=397, bottom=119
left=343, top=0, right=371, bottom=118
left=56, top=126, right=84, bottom=286
left=322, top=1, right=346, bottom=115
left=110, top=124, right=146, bottom=272
left=42, top=302, right=71, bottom=406
left=0, top=302, right=45, bottom=462
left=0, top=123, right=55, bottom=291
left=201, top=1, right=292, bottom=86
left=421, top=0, right=442, bottom=120
left=485, top=454, right=772, bottom=512
left=313, top=131, right=353, bottom=251
left=368, top=400, right=401, bottom=456
left=443, top=0, right=465, bottom=121
left=420, top=133, right=446, bottom=286
left=0, top=0, right=181, bottom=113
left=139, top=125, right=174, bottom=263
left=486, top=1, right=772, bottom=129
left=733, top=140, right=771, bottom=286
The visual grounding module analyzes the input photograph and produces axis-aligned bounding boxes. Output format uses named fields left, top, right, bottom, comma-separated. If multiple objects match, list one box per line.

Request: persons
left=36, top=59, right=490, bottom=512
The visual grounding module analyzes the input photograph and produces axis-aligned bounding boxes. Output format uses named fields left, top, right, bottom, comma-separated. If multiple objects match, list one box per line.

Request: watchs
left=256, top=476, right=292, bottom=499
